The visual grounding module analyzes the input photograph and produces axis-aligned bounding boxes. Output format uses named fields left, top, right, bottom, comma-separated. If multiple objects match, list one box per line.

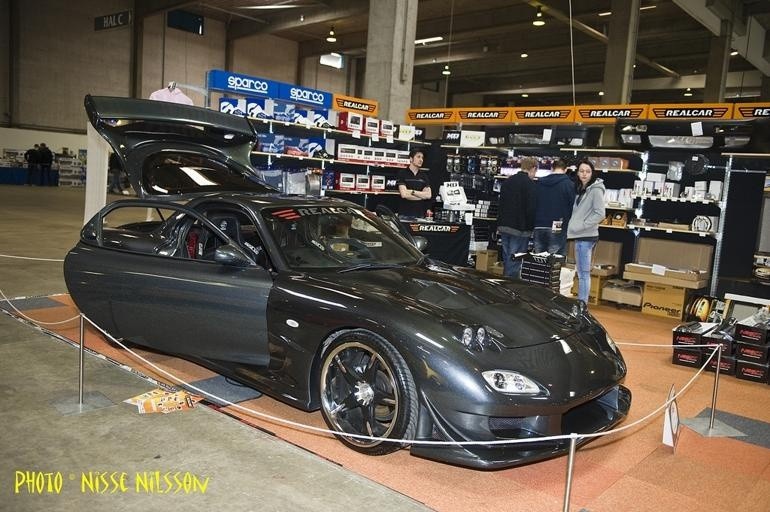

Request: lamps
left=730, top=48, right=739, bottom=56
left=325, top=24, right=337, bottom=44
left=530, top=5, right=546, bottom=27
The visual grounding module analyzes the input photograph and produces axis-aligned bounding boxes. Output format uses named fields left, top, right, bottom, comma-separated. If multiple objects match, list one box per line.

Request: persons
left=565, top=159, right=606, bottom=306
left=395, top=148, right=433, bottom=217
left=35, top=143, right=52, bottom=186
left=497, top=157, right=540, bottom=278
left=23, top=143, right=40, bottom=188
left=533, top=160, right=576, bottom=258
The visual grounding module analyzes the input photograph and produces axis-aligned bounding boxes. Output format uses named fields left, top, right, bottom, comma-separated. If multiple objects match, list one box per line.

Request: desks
left=0, top=167, right=58, bottom=187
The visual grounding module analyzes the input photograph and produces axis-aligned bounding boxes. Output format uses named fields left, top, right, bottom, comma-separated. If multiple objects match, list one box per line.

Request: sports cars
left=60, top=95, right=633, bottom=473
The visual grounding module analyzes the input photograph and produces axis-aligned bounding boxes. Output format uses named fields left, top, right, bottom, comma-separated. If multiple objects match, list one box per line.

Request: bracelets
left=411, top=190, right=415, bottom=195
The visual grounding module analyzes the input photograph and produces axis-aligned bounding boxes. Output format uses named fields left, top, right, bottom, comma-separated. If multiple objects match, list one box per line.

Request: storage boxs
left=638, top=279, right=693, bottom=321
left=672, top=312, right=770, bottom=384
